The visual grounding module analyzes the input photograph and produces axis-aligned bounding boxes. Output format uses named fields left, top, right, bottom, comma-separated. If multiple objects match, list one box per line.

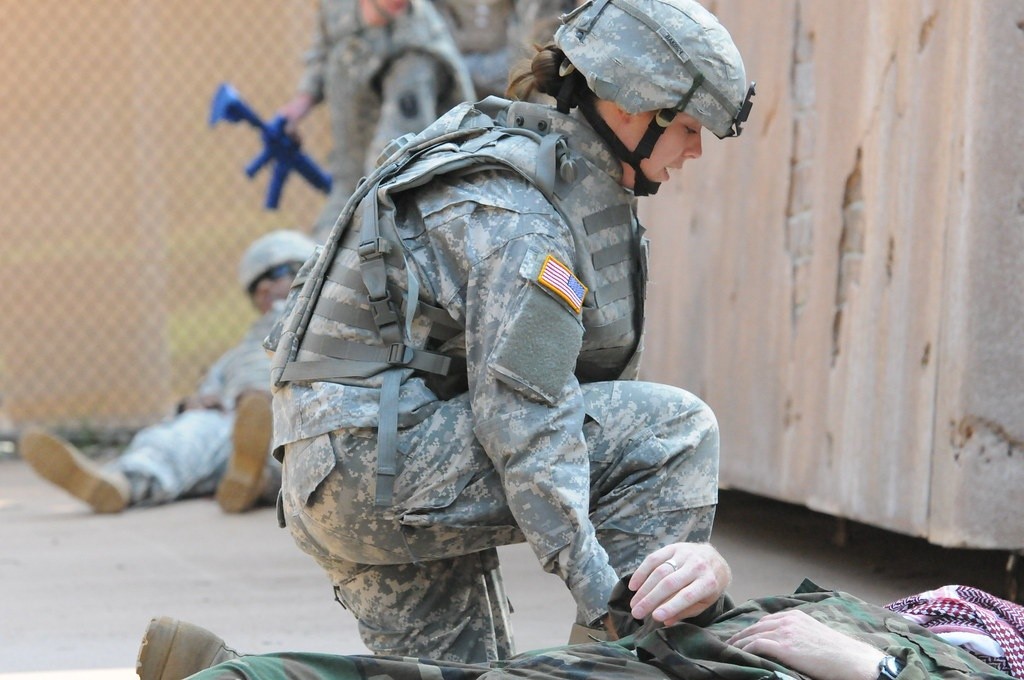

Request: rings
left=664, top=559, right=678, bottom=571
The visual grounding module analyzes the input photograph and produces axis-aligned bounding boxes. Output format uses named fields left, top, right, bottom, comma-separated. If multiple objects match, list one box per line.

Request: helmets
left=552, top=0, right=755, bottom=197
left=238, top=231, right=321, bottom=299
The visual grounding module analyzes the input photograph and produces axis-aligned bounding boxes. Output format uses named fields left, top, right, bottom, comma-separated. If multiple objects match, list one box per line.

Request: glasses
left=248, top=260, right=293, bottom=295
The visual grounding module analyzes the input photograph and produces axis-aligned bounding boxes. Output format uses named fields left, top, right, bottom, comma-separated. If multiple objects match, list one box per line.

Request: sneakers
left=17, top=428, right=129, bottom=515
left=214, top=390, right=274, bottom=515
left=135, top=617, right=224, bottom=680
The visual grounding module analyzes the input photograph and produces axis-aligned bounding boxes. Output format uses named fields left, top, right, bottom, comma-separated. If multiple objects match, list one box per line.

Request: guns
left=209, top=85, right=333, bottom=212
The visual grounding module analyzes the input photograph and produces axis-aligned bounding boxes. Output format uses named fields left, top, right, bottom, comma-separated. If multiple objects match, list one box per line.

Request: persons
left=273, top=0, right=756, bottom=661
left=17, top=225, right=315, bottom=514
left=276, top=0, right=579, bottom=248
left=135, top=583, right=1024, bottom=680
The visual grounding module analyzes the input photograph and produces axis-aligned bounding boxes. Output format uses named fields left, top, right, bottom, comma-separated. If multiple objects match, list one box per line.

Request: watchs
left=876, top=654, right=909, bottom=680
